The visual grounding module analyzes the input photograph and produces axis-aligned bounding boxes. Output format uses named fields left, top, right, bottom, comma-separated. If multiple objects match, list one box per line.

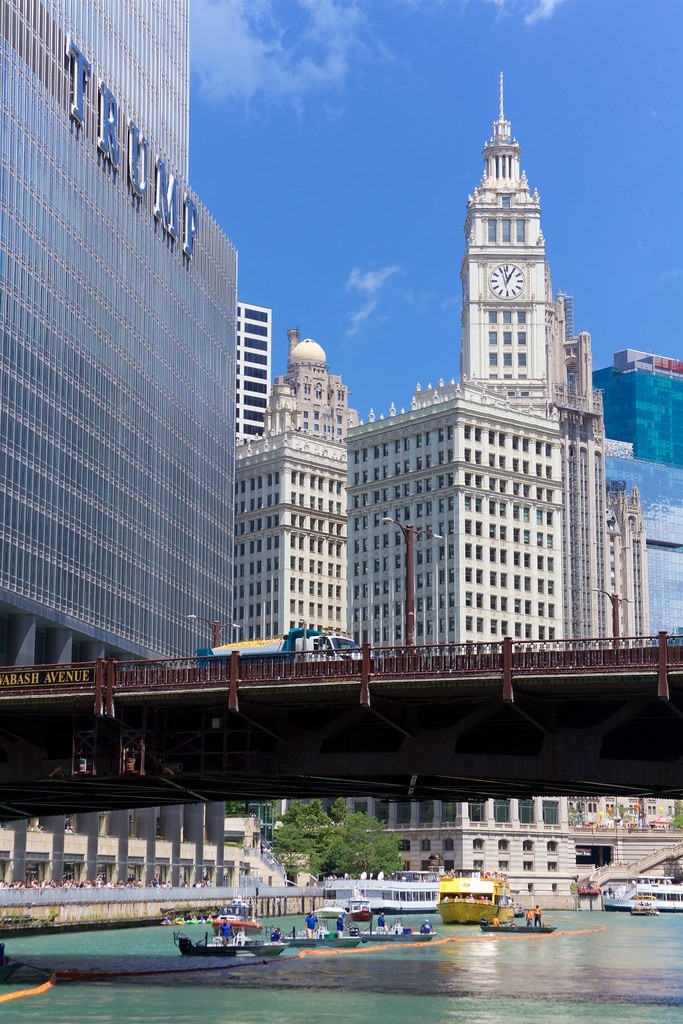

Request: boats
left=280, top=920, right=369, bottom=948
left=212, top=894, right=265, bottom=936
left=603, top=876, right=683, bottom=914
left=513, top=901, right=525, bottom=918
left=347, top=916, right=438, bottom=943
left=160, top=918, right=214, bottom=926
left=344, top=896, right=375, bottom=921
left=173, top=931, right=291, bottom=956
left=324, top=869, right=441, bottom=915
left=434, top=868, right=515, bottom=924
left=479, top=917, right=557, bottom=934
left=630, top=896, right=660, bottom=917
left=314, top=905, right=347, bottom=919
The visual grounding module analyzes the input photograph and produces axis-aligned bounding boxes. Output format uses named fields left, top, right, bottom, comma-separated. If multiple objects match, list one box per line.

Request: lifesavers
left=510, top=922, right=518, bottom=927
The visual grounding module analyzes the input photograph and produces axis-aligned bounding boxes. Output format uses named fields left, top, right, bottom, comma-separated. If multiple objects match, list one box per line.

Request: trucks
left=195, top=618, right=363, bottom=668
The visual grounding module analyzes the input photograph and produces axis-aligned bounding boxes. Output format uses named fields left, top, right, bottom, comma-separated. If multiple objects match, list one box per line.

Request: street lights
left=382, top=517, right=442, bottom=653
left=187, top=615, right=240, bottom=649
left=591, top=587, right=635, bottom=649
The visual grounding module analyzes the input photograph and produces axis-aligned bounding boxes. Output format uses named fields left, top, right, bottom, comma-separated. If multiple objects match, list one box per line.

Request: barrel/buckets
left=403, top=927, right=414, bottom=934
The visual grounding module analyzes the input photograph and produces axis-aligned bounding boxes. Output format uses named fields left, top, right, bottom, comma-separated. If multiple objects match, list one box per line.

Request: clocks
left=489, top=264, right=526, bottom=300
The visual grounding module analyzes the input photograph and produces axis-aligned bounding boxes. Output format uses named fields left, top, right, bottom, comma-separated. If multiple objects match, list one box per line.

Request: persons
left=163, top=908, right=218, bottom=924
left=419, top=919, right=434, bottom=933
left=336, top=915, right=350, bottom=938
left=0, top=878, right=212, bottom=892
left=443, top=872, right=453, bottom=878
left=534, top=904, right=542, bottom=927
left=493, top=916, right=500, bottom=926
left=442, top=894, right=474, bottom=902
left=377, top=912, right=389, bottom=935
left=270, top=927, right=283, bottom=941
left=220, top=919, right=235, bottom=947
left=305, top=911, right=320, bottom=939
left=507, top=897, right=512, bottom=906
left=638, top=902, right=651, bottom=906
left=27, top=825, right=45, bottom=833
left=64, top=825, right=74, bottom=834
left=526, top=908, right=534, bottom=927
left=481, top=870, right=509, bottom=881
